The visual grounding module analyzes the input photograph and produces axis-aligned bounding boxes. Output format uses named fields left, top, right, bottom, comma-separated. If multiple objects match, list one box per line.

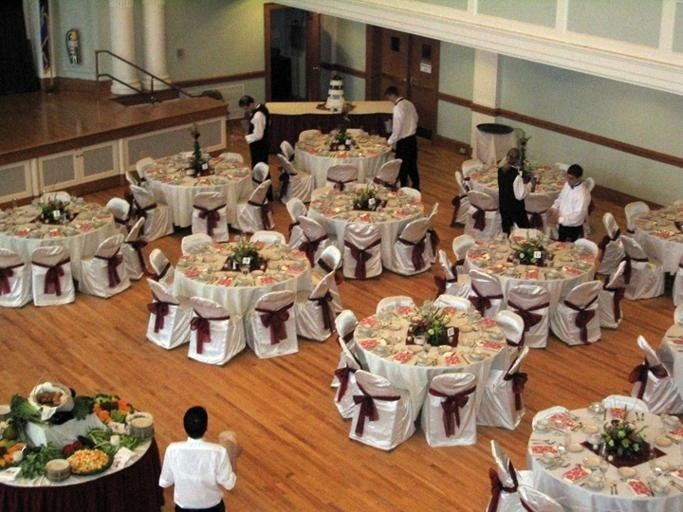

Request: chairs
left=201, top=87, right=233, bottom=132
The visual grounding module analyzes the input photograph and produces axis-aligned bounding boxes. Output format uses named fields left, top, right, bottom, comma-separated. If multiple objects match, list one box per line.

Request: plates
left=0, top=404, right=10, bottom=420
left=45, top=458, right=70, bottom=481
left=0, top=205, right=110, bottom=239
left=65, top=452, right=112, bottom=475
left=179, top=242, right=307, bottom=287
left=532, top=403, right=683, bottom=499
left=358, top=301, right=504, bottom=366
left=126, top=412, right=154, bottom=439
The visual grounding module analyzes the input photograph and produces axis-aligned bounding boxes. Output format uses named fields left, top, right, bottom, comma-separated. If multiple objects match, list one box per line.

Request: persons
left=228, top=96, right=273, bottom=206
left=158, top=405, right=242, bottom=511
left=548, top=164, right=591, bottom=242
left=384, top=85, right=420, bottom=193
left=496, top=149, right=542, bottom=238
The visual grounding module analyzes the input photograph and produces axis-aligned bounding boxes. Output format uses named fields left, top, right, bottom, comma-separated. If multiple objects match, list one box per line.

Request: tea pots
left=219, top=430, right=237, bottom=465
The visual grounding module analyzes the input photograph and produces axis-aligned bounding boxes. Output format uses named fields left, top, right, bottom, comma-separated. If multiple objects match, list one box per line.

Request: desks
left=472, top=123, right=515, bottom=165
left=260, top=99, right=396, bottom=144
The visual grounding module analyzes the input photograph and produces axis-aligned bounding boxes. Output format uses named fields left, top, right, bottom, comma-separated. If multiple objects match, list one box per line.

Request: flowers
left=517, top=132, right=531, bottom=167
left=185, top=119, right=204, bottom=163
left=334, top=100, right=356, bottom=142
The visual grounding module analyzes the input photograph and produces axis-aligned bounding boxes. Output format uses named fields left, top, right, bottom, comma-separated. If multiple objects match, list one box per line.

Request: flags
left=38, top=0, right=51, bottom=73
